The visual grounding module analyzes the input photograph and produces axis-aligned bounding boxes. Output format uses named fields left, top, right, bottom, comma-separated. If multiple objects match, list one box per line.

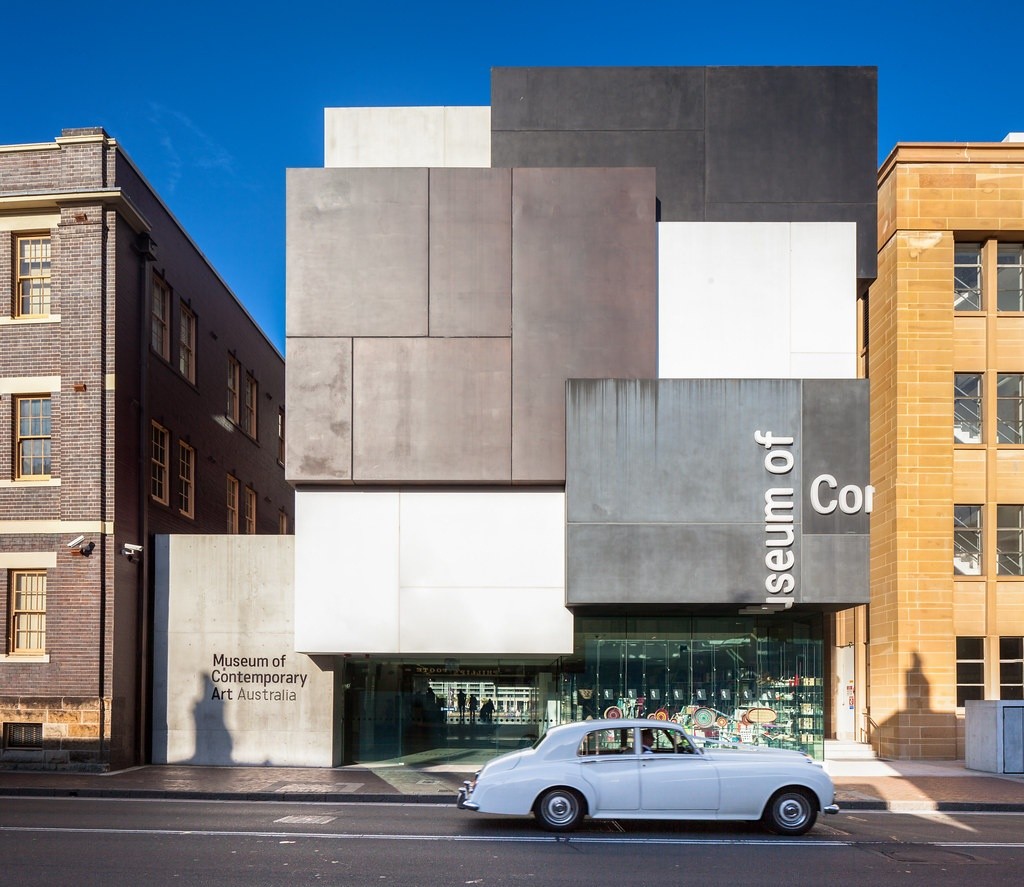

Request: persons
left=413, top=685, right=496, bottom=726
left=641, top=730, right=657, bottom=754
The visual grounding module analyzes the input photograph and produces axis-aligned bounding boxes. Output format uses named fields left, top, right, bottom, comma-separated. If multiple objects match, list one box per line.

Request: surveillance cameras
left=67, top=535, right=85, bottom=548
left=124, top=543, right=144, bottom=552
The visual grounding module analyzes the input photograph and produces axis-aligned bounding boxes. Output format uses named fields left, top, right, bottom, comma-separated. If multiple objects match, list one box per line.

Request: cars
left=456, top=719, right=840, bottom=835
